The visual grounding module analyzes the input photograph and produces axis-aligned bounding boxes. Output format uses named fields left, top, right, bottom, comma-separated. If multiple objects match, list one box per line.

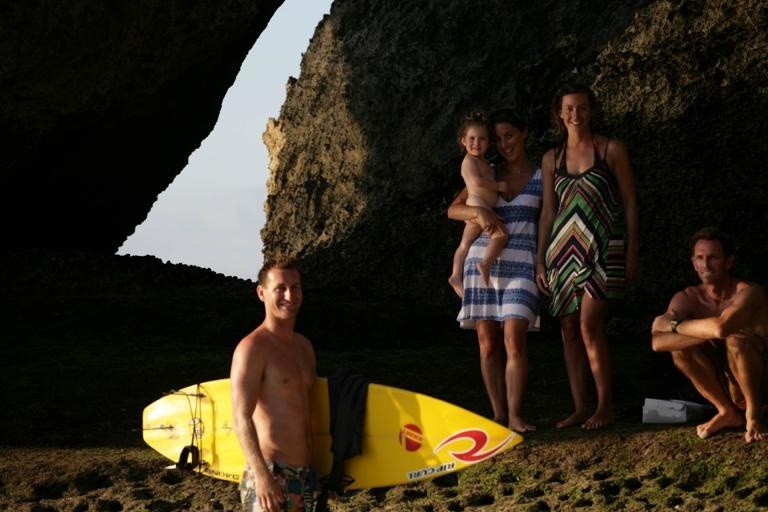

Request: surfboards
left=142, top=379, right=524, bottom=492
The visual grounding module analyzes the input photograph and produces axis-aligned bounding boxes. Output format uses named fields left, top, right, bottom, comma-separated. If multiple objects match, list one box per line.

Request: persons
left=651, top=227, right=768, bottom=443
left=532, top=84, right=637, bottom=432
left=229, top=256, right=317, bottom=512
left=448, top=111, right=507, bottom=299
left=446, top=105, right=542, bottom=433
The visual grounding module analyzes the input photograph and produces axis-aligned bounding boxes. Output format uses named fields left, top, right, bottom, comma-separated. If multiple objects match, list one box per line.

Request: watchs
left=670, top=316, right=685, bottom=332
